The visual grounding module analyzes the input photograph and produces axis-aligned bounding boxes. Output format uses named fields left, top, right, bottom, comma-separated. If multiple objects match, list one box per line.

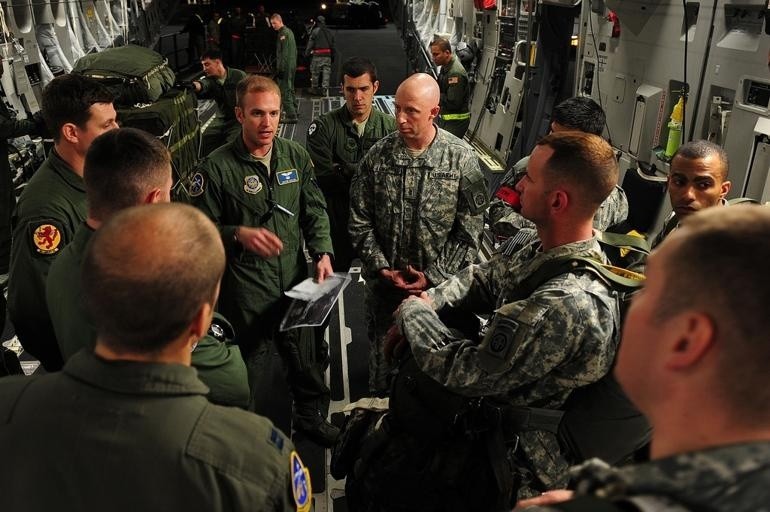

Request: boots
left=281, top=88, right=327, bottom=124
left=294, top=391, right=341, bottom=444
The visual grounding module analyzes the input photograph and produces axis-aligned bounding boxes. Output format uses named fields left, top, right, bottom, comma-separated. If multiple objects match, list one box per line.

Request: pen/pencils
left=265, top=199, right=295, bottom=218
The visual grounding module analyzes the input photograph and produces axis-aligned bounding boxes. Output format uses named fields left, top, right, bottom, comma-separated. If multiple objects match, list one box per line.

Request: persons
left=0, top=204, right=317, bottom=511
left=174, top=50, right=250, bottom=156
left=367, top=131, right=626, bottom=510
left=505, top=202, right=769, bottom=510
left=178, top=72, right=340, bottom=495
left=265, top=11, right=297, bottom=126
left=35, top=124, right=257, bottom=417
left=429, top=35, right=472, bottom=141
left=647, top=139, right=734, bottom=254
left=303, top=13, right=334, bottom=96
left=481, top=93, right=629, bottom=252
left=5, top=72, right=130, bottom=370
left=336, top=72, right=486, bottom=397
